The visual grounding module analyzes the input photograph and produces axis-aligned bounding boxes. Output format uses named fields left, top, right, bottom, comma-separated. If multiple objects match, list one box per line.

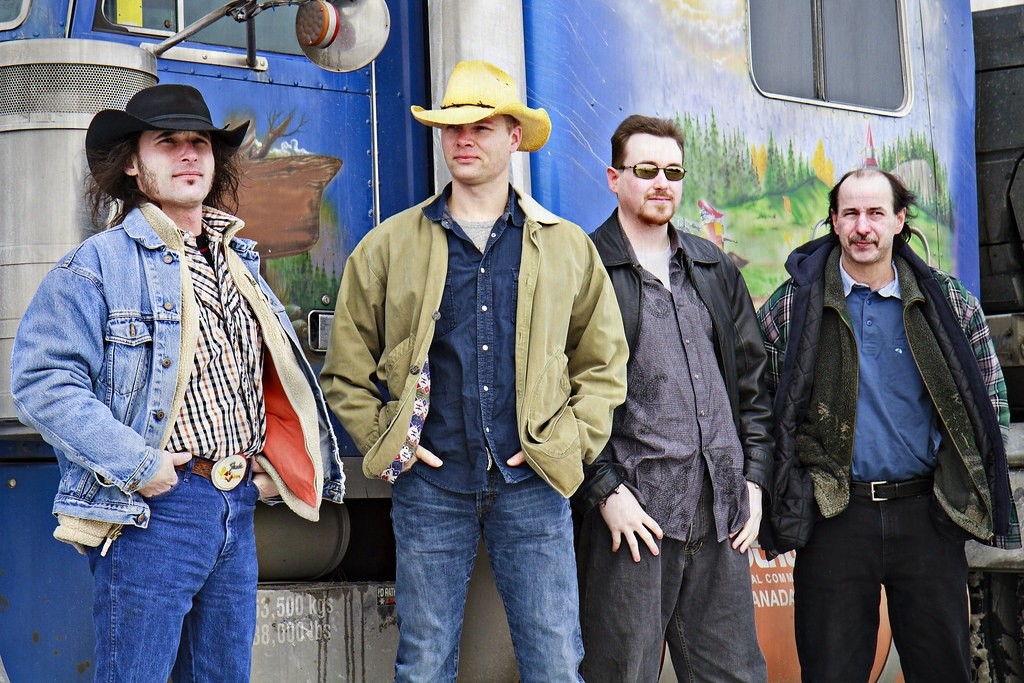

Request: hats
left=85, top=84, right=251, bottom=199
left=411, top=60, right=552, bottom=151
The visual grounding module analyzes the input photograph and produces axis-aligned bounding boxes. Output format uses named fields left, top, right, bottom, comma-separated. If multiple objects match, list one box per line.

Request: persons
left=754, top=168, right=1024, bottom=683
left=8, top=83, right=346, bottom=683
left=566, top=109, right=772, bottom=683
left=318, top=60, right=632, bottom=683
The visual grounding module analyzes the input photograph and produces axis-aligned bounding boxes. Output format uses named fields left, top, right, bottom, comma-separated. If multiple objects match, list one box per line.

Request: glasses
left=618, top=163, right=687, bottom=181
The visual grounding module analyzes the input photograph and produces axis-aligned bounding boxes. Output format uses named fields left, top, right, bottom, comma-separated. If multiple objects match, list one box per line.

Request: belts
left=175, top=455, right=252, bottom=491
left=849, top=477, right=933, bottom=501
